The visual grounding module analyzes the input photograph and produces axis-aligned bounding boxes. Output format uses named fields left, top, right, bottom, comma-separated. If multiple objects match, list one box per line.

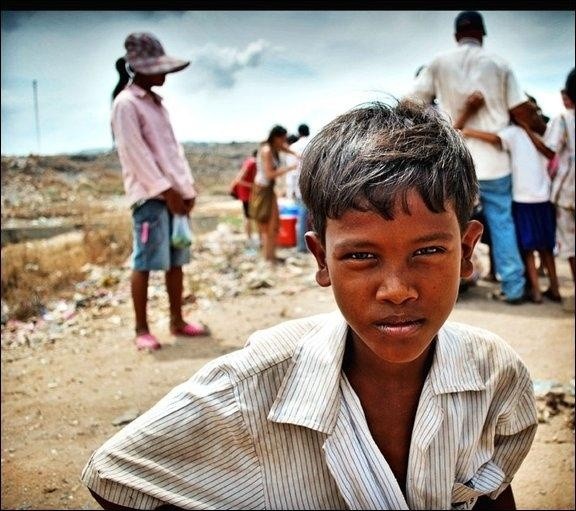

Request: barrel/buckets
left=275, top=214, right=298, bottom=248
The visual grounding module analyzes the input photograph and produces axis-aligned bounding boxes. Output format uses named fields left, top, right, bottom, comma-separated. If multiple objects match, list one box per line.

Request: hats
left=125, top=32, right=190, bottom=75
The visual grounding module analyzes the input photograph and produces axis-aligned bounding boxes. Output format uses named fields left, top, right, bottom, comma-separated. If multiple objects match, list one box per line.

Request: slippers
left=134, top=334, right=160, bottom=350
left=171, top=322, right=204, bottom=335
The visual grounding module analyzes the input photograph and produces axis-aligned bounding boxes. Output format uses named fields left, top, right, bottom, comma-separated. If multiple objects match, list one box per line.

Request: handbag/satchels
left=247, top=185, right=272, bottom=222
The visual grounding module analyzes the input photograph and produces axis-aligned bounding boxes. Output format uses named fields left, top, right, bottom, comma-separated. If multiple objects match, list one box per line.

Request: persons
left=251, top=124, right=302, bottom=266
left=414, top=64, right=576, bottom=303
left=404, top=10, right=547, bottom=305
left=107, top=30, right=211, bottom=351
left=80, top=96, right=538, bottom=510
left=229, top=148, right=263, bottom=251
left=286, top=124, right=311, bottom=256
